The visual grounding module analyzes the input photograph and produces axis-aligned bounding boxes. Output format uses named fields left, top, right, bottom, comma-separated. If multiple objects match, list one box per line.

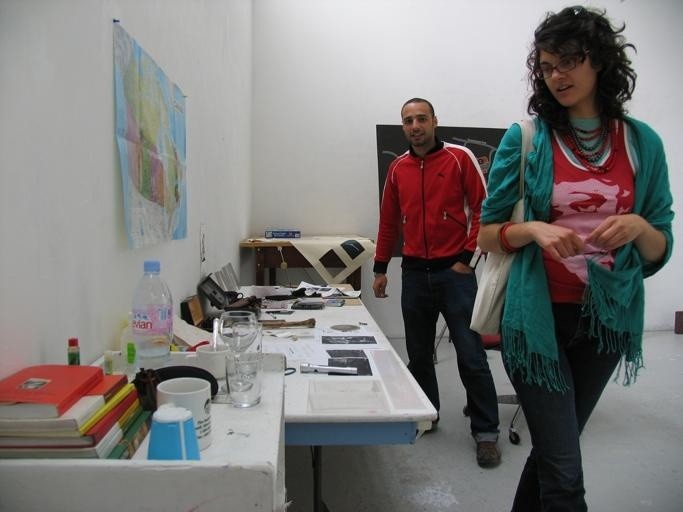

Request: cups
left=184, top=344, right=232, bottom=381
left=225, top=351, right=261, bottom=407
left=155, top=377, right=211, bottom=451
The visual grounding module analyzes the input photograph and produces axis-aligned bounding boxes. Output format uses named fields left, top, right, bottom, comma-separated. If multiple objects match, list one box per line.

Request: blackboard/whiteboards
left=376, top=124, right=510, bottom=257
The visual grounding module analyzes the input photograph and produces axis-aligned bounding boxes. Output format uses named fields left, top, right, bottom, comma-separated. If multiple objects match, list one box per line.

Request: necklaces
left=561, top=118, right=618, bottom=174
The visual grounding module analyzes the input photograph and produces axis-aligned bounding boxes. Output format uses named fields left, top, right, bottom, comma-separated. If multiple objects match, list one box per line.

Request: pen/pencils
left=265, top=311, right=295, bottom=319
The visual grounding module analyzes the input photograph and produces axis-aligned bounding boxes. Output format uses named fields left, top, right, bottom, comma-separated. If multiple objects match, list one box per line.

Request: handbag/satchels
left=468, top=198, right=527, bottom=336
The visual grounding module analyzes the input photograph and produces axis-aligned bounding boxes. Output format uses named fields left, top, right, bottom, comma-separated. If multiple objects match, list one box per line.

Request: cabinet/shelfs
left=0, top=348, right=287, bottom=512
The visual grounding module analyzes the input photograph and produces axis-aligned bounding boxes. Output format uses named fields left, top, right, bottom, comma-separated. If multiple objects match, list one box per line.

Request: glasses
left=533, top=48, right=592, bottom=81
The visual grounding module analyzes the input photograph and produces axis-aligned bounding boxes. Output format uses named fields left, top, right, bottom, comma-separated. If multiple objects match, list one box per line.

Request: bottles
left=131, top=259, right=173, bottom=370
left=67, top=336, right=80, bottom=365
left=103, top=350, right=113, bottom=375
left=147, top=403, right=200, bottom=460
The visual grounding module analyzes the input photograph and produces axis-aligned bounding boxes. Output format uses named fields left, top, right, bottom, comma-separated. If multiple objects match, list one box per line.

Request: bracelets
left=496, top=221, right=516, bottom=254
left=373, top=272, right=385, bottom=277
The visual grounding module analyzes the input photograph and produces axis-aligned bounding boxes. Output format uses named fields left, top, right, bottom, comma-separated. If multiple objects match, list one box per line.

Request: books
left=0, top=365, right=152, bottom=459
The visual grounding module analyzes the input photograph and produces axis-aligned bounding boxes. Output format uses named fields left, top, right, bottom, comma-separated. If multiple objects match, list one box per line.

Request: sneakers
left=476, top=441, right=501, bottom=468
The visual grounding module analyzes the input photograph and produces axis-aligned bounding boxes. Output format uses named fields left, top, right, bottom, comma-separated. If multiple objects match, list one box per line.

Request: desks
left=173, top=284, right=441, bottom=512
left=239, top=235, right=376, bottom=299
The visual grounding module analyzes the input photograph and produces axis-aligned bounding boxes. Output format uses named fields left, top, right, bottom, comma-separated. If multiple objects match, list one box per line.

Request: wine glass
left=217, top=311, right=258, bottom=392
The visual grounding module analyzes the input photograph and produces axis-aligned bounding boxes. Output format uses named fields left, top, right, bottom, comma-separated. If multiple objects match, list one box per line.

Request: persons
left=373, top=98, right=500, bottom=467
left=476, top=5, right=674, bottom=512
